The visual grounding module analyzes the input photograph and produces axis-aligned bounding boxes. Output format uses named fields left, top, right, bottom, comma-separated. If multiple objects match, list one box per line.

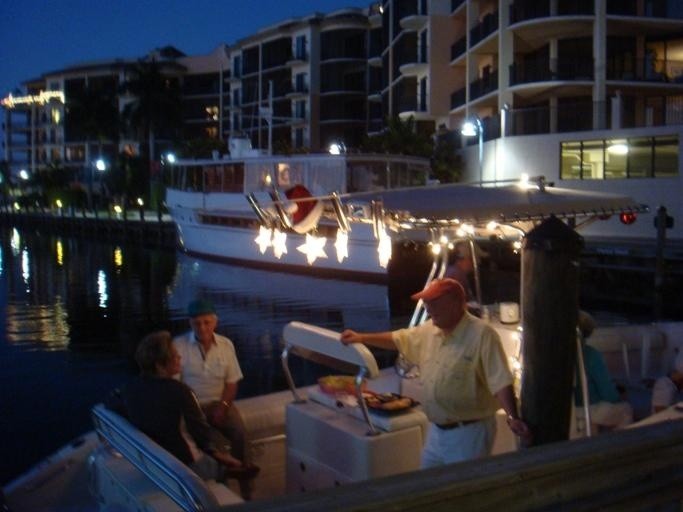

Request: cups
left=499, top=302, right=520, bottom=323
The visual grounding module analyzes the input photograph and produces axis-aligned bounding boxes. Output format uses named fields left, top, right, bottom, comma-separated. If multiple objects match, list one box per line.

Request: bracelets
left=221, top=398, right=231, bottom=408
left=506, top=415, right=521, bottom=424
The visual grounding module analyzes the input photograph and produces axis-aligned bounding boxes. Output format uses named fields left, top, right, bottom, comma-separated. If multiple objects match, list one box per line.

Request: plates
left=316, top=373, right=367, bottom=393
left=356, top=391, right=420, bottom=416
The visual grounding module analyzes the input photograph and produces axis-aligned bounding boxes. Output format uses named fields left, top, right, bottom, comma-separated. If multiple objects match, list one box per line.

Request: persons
left=440, top=240, right=490, bottom=320
left=339, top=277, right=534, bottom=468
left=171, top=295, right=257, bottom=501
left=651, top=350, right=682, bottom=416
left=570, top=305, right=634, bottom=436
left=118, top=330, right=261, bottom=481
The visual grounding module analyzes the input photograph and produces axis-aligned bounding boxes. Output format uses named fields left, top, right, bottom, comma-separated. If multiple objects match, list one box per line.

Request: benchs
left=92, top=404, right=246, bottom=512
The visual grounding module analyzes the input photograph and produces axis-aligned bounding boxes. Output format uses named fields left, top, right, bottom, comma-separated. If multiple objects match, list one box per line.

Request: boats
left=164, top=137, right=520, bottom=281
left=2, top=184, right=683, bottom=512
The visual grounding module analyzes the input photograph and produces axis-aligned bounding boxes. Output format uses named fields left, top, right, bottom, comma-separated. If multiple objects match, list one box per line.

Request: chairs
left=278, top=318, right=429, bottom=449
left=566, top=327, right=598, bottom=444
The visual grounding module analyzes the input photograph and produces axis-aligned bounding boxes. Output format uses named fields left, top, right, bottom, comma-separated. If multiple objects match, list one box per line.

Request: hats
left=185, top=298, right=216, bottom=317
left=410, top=278, right=467, bottom=304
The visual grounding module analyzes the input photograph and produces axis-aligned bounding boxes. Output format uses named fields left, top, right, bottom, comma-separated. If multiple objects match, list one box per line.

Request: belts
left=435, top=418, right=482, bottom=430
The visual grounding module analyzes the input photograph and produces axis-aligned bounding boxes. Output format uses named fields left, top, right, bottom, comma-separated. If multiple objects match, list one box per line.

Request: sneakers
left=223, top=463, right=262, bottom=480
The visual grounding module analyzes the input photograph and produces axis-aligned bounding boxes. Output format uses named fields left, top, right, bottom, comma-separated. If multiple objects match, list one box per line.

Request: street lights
left=461, top=113, right=484, bottom=186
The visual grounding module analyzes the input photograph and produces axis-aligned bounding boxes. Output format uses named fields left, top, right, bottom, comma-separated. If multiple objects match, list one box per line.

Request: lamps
left=253, top=214, right=524, bottom=270
